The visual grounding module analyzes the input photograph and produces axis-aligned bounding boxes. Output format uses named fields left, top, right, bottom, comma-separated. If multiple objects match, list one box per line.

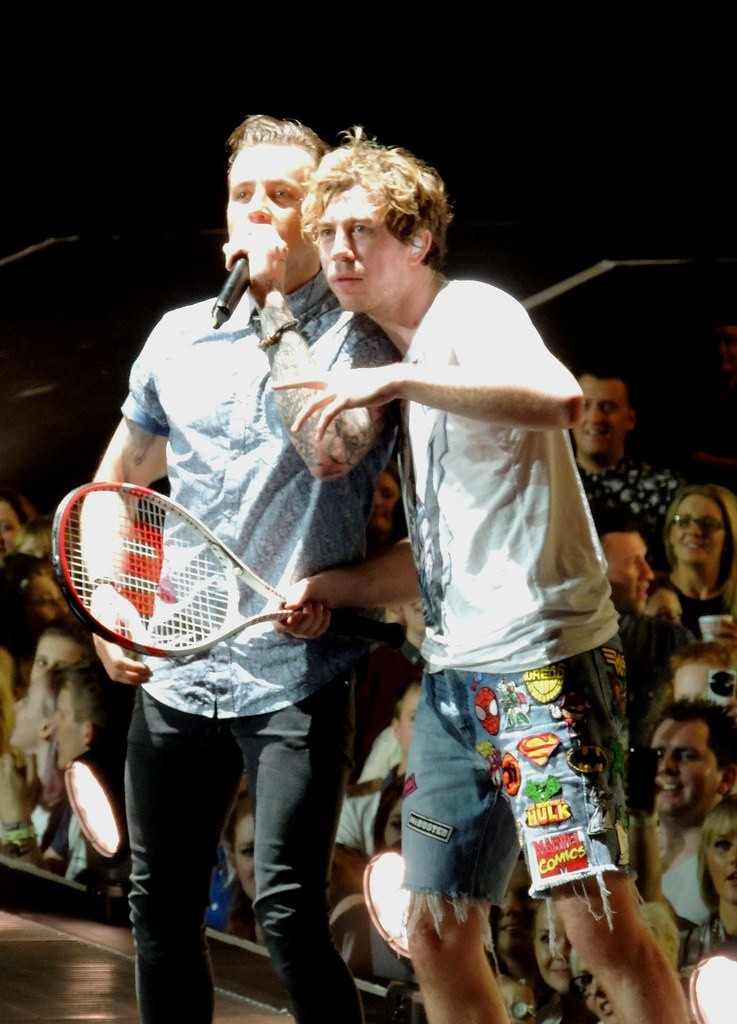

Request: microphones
left=211, top=254, right=252, bottom=330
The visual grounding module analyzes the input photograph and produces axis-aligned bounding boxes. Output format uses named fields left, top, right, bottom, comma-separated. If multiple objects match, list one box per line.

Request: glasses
left=673, top=512, right=726, bottom=533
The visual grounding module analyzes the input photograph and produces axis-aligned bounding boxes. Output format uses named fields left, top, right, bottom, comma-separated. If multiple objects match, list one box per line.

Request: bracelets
left=2, top=840, right=36, bottom=859
left=6, top=827, right=35, bottom=841
left=85, top=576, right=116, bottom=597
left=259, top=320, right=301, bottom=351
left=629, top=812, right=659, bottom=826
left=4, top=820, right=31, bottom=831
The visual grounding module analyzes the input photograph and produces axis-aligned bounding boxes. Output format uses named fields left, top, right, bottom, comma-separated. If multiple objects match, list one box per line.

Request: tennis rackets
left=50, top=481, right=407, bottom=660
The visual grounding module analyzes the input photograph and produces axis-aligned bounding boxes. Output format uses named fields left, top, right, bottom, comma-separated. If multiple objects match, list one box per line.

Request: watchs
left=508, top=1002, right=537, bottom=1020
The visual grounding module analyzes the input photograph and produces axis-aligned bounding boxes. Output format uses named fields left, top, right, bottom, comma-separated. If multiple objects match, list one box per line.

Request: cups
left=698, top=614, right=733, bottom=643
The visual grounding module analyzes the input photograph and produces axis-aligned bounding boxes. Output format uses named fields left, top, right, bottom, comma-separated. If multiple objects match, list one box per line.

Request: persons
left=0, top=357, right=736, bottom=1023
left=76, top=116, right=401, bottom=1024
left=271, top=124, right=692, bottom=1024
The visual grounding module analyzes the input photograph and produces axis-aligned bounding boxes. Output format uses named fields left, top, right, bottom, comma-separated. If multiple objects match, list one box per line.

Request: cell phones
left=627, top=744, right=662, bottom=817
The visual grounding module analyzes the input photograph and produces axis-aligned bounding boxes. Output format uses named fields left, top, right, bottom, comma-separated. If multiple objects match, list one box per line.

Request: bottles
left=6, top=820, right=53, bottom=875
